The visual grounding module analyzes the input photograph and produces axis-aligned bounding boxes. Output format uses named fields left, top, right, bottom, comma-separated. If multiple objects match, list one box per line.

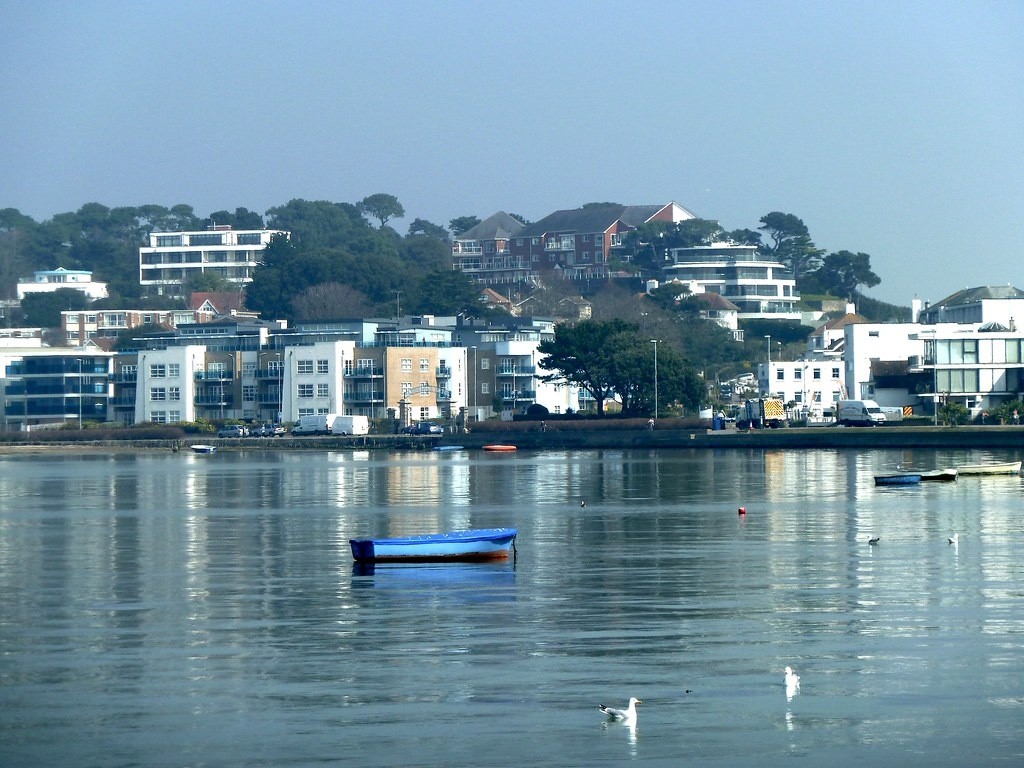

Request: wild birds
left=781, top=666, right=802, bottom=684
left=579, top=500, right=587, bottom=508
left=948, top=533, right=960, bottom=543
left=593, top=697, right=641, bottom=719
left=866, top=534, right=880, bottom=544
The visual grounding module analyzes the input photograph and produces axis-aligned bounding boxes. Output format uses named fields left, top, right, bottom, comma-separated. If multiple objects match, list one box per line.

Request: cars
left=217, top=425, right=249, bottom=438
left=401, top=421, right=441, bottom=434
left=736, top=372, right=754, bottom=380
left=718, top=385, right=732, bottom=400
left=251, top=423, right=287, bottom=437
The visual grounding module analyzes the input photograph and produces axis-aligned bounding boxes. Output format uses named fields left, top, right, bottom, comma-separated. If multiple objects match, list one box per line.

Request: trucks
left=836, top=400, right=887, bottom=427
left=736, top=397, right=784, bottom=430
left=291, top=414, right=342, bottom=437
left=331, top=416, right=368, bottom=436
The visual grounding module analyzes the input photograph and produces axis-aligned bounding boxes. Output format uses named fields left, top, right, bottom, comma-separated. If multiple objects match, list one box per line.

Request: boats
left=349, top=528, right=518, bottom=562
left=191, top=444, right=217, bottom=453
left=873, top=474, right=922, bottom=486
left=432, top=445, right=464, bottom=451
left=897, top=468, right=958, bottom=481
left=482, top=444, right=516, bottom=451
left=957, top=460, right=1022, bottom=475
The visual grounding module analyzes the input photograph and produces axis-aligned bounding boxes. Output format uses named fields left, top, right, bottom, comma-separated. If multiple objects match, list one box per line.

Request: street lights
left=76, top=358, right=82, bottom=430
left=276, top=353, right=280, bottom=423
left=471, top=345, right=478, bottom=422
left=764, top=334, right=771, bottom=362
left=651, top=339, right=658, bottom=419
